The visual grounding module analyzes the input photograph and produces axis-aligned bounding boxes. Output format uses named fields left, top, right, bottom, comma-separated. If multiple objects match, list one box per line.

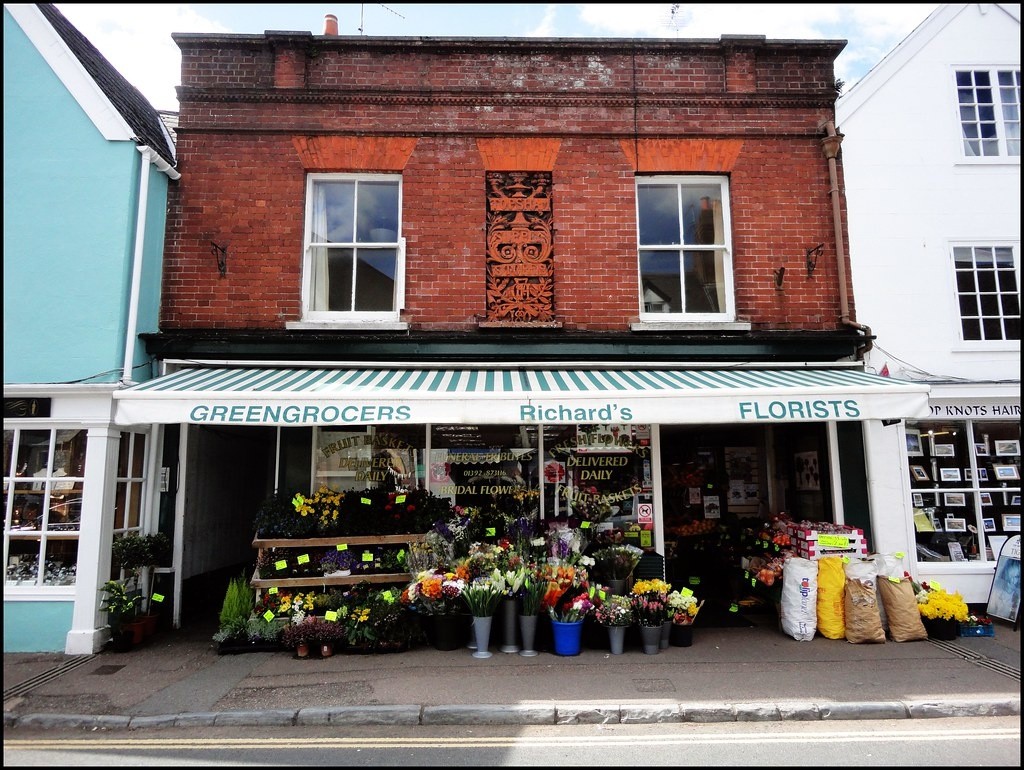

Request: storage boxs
left=634, top=552, right=664, bottom=581
left=787, top=525, right=868, bottom=558
left=960, top=623, right=994, bottom=637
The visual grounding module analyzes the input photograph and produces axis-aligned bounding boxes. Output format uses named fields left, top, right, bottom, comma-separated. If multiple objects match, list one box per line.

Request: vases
left=608, top=579, right=626, bottom=596
left=467, top=621, right=478, bottom=649
left=436, top=616, right=457, bottom=651
left=670, top=623, right=693, bottom=647
left=641, top=625, right=662, bottom=654
left=603, top=625, right=630, bottom=655
left=122, top=620, right=144, bottom=644
left=139, top=616, right=156, bottom=634
left=660, top=621, right=672, bottom=648
left=321, top=643, right=333, bottom=657
left=519, top=615, right=539, bottom=657
left=499, top=601, right=521, bottom=653
left=472, top=616, right=493, bottom=660
left=297, top=644, right=310, bottom=656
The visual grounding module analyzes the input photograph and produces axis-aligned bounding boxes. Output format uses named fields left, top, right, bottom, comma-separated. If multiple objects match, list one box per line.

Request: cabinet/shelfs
left=250, top=530, right=426, bottom=621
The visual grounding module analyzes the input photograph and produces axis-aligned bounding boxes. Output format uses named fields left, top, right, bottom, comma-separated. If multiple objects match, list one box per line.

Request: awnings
left=112, top=366, right=934, bottom=427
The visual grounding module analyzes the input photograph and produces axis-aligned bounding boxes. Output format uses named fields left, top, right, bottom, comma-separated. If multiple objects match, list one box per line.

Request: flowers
left=99, top=530, right=170, bottom=635
left=665, top=511, right=843, bottom=586
left=213, top=485, right=705, bottom=648
left=904, top=571, right=992, bottom=625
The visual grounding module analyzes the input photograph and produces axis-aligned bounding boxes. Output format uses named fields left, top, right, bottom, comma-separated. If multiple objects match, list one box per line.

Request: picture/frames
left=913, top=494, right=924, bottom=506
left=983, top=518, right=996, bottom=532
left=979, top=468, right=989, bottom=481
left=1011, top=496, right=1021, bottom=505
left=910, top=465, right=930, bottom=481
left=940, top=468, right=962, bottom=481
left=944, top=493, right=965, bottom=506
left=935, top=444, right=954, bottom=456
left=905, top=429, right=924, bottom=456
left=980, top=493, right=993, bottom=506
left=964, top=468, right=980, bottom=481
left=993, top=465, right=1021, bottom=480
left=974, top=443, right=990, bottom=456
left=945, top=517, right=966, bottom=532
left=932, top=518, right=943, bottom=532
left=995, top=440, right=1021, bottom=456
left=1001, top=514, right=1022, bottom=531
